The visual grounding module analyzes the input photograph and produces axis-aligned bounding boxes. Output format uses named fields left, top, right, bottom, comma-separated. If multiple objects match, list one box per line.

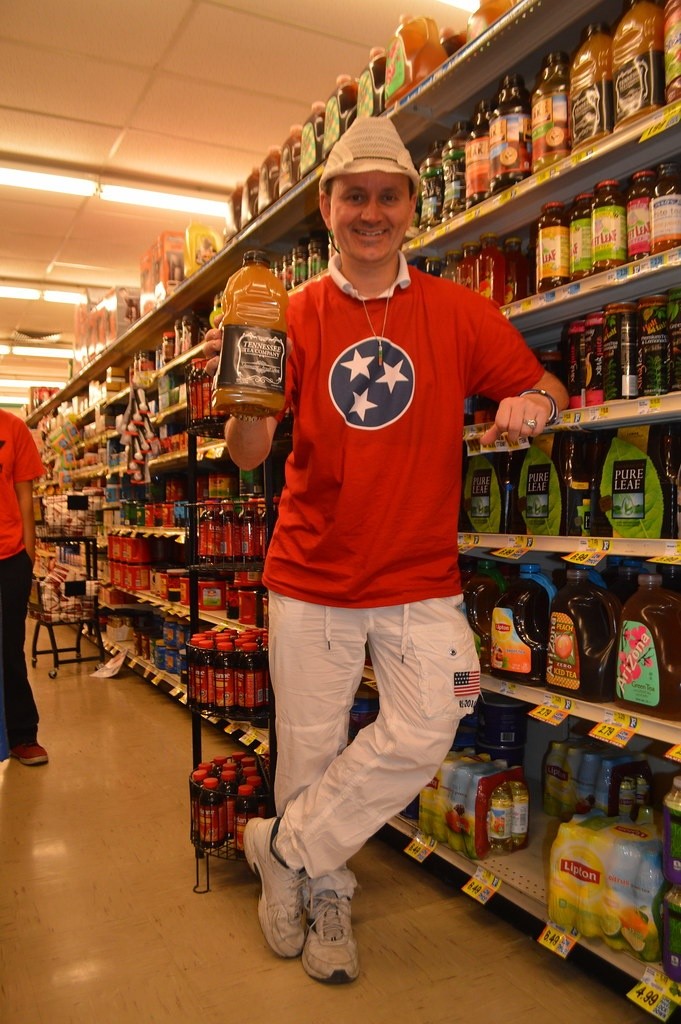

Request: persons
left=201, top=114, right=569, bottom=983
left=0, top=408, right=49, bottom=764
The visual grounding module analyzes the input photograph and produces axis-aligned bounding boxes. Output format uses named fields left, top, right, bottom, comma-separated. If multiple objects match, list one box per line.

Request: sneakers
left=9, top=740, right=49, bottom=764
left=301, top=869, right=359, bottom=983
left=244, top=817, right=308, bottom=956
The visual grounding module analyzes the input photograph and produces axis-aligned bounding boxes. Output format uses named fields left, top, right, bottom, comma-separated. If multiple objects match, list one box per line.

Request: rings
left=523, top=419, right=537, bottom=429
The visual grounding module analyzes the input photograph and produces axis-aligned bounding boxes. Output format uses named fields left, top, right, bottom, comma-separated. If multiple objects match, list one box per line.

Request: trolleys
left=25, top=523, right=105, bottom=678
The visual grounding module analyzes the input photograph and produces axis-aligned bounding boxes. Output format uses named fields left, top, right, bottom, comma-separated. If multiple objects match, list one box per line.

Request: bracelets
left=519, top=389, right=558, bottom=427
left=232, top=413, right=262, bottom=422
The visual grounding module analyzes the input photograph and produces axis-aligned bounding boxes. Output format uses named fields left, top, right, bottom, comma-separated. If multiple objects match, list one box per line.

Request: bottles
left=457, top=556, right=681, bottom=721
left=258, top=146, right=288, bottom=215
left=133, top=349, right=155, bottom=375
left=274, top=230, right=339, bottom=291
left=419, top=737, right=681, bottom=982
left=162, top=313, right=208, bottom=363
left=278, top=74, right=358, bottom=198
left=198, top=496, right=286, bottom=566
left=184, top=218, right=223, bottom=277
left=417, top=0, right=681, bottom=306
left=223, top=166, right=270, bottom=243
left=133, top=617, right=190, bottom=676
left=357, top=47, right=396, bottom=117
left=189, top=357, right=207, bottom=426
left=191, top=752, right=267, bottom=858
left=209, top=292, right=224, bottom=329
left=211, top=250, right=288, bottom=417
left=107, top=615, right=131, bottom=628
left=456, top=421, right=681, bottom=539
left=191, top=628, right=268, bottom=712
left=202, top=361, right=228, bottom=424
left=384, top=11, right=449, bottom=108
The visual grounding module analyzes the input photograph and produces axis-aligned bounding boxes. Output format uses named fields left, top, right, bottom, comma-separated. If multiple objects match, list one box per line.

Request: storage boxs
left=22, top=366, right=135, bottom=605
left=73, top=231, right=192, bottom=371
left=107, top=625, right=134, bottom=641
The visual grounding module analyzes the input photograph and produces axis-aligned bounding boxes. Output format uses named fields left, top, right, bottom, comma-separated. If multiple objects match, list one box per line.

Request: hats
left=319, top=117, right=420, bottom=194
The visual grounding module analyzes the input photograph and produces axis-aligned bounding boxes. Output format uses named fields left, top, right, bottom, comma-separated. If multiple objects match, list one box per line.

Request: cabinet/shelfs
left=0, top=0, right=681, bottom=1024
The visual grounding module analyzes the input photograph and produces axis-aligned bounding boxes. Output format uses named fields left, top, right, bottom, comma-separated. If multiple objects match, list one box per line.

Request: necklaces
left=359, top=272, right=396, bottom=366
